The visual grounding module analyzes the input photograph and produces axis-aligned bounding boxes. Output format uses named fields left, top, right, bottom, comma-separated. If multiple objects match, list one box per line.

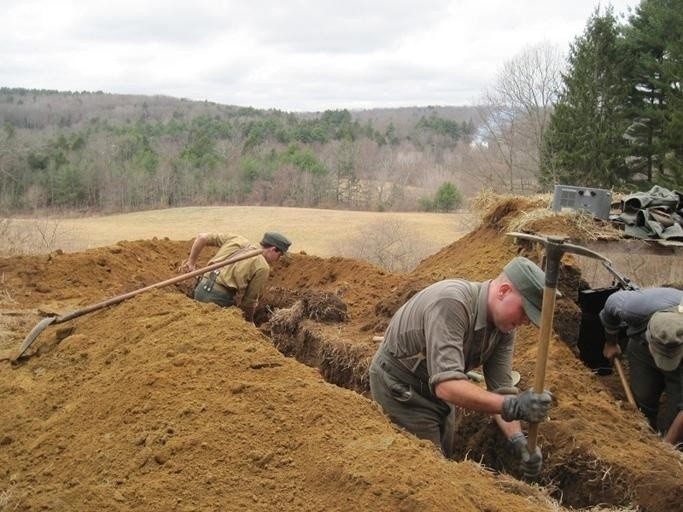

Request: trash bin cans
left=578, top=288, right=622, bottom=375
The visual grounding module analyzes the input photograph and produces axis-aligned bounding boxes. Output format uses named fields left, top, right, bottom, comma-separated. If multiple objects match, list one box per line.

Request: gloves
left=500, top=387, right=552, bottom=424
left=506, top=431, right=543, bottom=478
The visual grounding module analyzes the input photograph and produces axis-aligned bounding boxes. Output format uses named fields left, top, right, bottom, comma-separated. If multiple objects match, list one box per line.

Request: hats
left=645, top=308, right=683, bottom=371
left=263, top=231, right=292, bottom=258
left=503, top=255, right=562, bottom=329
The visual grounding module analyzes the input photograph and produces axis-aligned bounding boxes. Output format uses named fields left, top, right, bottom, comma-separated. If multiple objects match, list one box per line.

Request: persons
left=599, top=287, right=683, bottom=453
left=182, top=231, right=292, bottom=329
left=368, top=256, right=561, bottom=484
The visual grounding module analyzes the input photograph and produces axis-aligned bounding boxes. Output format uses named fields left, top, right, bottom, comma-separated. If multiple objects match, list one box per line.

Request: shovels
left=13, top=249, right=263, bottom=361
left=372, top=336, right=522, bottom=394
